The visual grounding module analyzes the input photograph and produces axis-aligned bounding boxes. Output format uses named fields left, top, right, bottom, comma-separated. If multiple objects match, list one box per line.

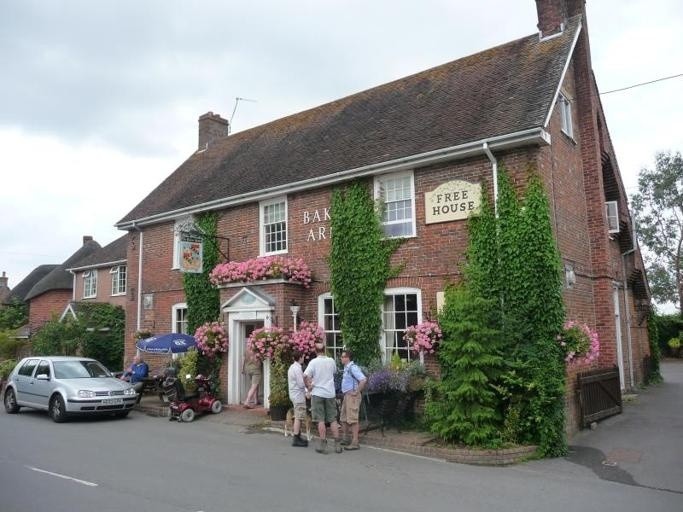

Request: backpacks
left=349, top=364, right=369, bottom=392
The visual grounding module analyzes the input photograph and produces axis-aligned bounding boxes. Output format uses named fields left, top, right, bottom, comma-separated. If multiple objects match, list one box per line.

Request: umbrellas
left=136, top=333, right=198, bottom=360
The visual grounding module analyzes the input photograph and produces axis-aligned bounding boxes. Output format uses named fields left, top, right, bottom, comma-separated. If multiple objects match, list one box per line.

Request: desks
left=136, top=376, right=165, bottom=403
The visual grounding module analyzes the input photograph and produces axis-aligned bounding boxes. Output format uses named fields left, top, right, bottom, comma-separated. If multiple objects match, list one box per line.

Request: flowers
left=246, top=318, right=326, bottom=366
left=401, top=318, right=442, bottom=354
left=557, top=322, right=599, bottom=367
left=207, top=254, right=314, bottom=290
left=193, top=321, right=229, bottom=356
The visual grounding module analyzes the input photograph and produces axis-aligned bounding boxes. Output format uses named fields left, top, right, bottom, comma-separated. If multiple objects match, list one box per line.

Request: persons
left=304, top=376, right=341, bottom=429
left=303, top=343, right=342, bottom=453
left=339, top=350, right=368, bottom=450
left=241, top=351, right=262, bottom=409
left=287, top=351, right=311, bottom=447
left=120, top=353, right=148, bottom=406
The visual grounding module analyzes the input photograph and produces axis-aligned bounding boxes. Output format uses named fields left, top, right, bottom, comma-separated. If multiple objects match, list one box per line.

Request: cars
left=2, top=355, right=140, bottom=423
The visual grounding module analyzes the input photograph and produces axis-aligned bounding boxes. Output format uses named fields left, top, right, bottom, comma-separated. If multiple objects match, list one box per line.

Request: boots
left=334, top=439, right=343, bottom=453
left=315, top=440, right=328, bottom=454
left=292, top=433, right=308, bottom=447
left=341, top=438, right=359, bottom=450
